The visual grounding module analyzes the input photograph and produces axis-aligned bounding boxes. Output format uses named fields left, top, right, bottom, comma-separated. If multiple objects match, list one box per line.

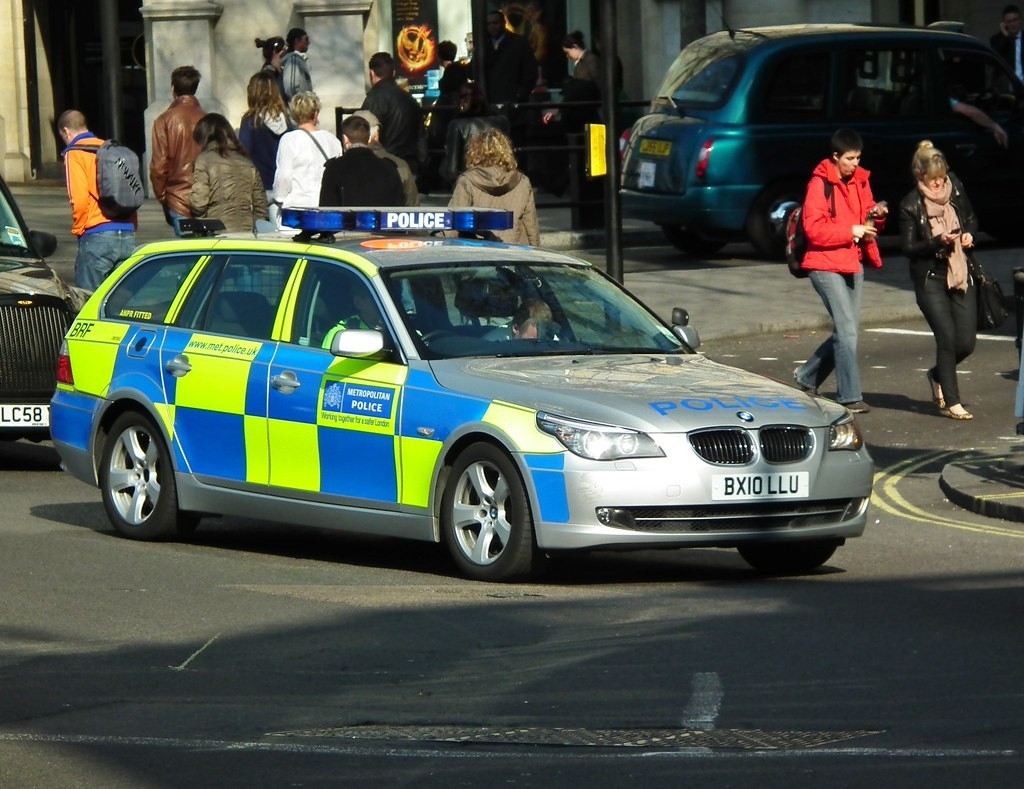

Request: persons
left=190, top=112, right=268, bottom=235
left=901, top=140, right=980, bottom=418
left=238, top=12, right=626, bottom=252
left=150, top=66, right=206, bottom=239
left=58, top=110, right=138, bottom=294
left=932, top=70, right=1006, bottom=145
left=991, top=6, right=1024, bottom=90
left=479, top=299, right=561, bottom=342
left=322, top=279, right=427, bottom=353
left=793, top=129, right=888, bottom=413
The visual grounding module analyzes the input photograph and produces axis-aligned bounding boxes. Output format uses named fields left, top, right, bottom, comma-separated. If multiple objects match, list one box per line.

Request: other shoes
left=927, top=369, right=946, bottom=409
left=945, top=403, right=974, bottom=419
left=793, top=367, right=822, bottom=398
left=841, top=401, right=871, bottom=413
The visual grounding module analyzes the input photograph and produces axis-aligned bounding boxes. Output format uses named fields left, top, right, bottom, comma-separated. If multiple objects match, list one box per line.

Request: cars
left=49, top=204, right=874, bottom=583
left=0, top=175, right=89, bottom=464
left=618, top=20, right=1024, bottom=254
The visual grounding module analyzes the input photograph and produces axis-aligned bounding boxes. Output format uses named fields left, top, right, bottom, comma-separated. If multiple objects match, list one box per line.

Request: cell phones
left=950, top=228, right=962, bottom=234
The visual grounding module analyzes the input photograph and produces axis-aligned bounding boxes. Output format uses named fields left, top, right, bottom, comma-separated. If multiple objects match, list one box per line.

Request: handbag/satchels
left=969, top=264, right=1010, bottom=329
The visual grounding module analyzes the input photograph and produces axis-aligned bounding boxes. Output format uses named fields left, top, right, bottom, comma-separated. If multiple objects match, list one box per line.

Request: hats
left=352, top=110, right=381, bottom=128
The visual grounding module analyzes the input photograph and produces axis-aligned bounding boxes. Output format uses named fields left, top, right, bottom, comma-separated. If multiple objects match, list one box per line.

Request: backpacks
left=783, top=181, right=833, bottom=278
left=61, top=138, right=144, bottom=219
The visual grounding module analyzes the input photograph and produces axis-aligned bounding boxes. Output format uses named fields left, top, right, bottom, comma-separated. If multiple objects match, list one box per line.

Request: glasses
left=487, top=20, right=499, bottom=26
left=452, top=95, right=471, bottom=104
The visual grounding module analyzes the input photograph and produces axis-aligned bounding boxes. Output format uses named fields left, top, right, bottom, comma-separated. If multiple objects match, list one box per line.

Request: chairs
left=205, top=277, right=522, bottom=357
